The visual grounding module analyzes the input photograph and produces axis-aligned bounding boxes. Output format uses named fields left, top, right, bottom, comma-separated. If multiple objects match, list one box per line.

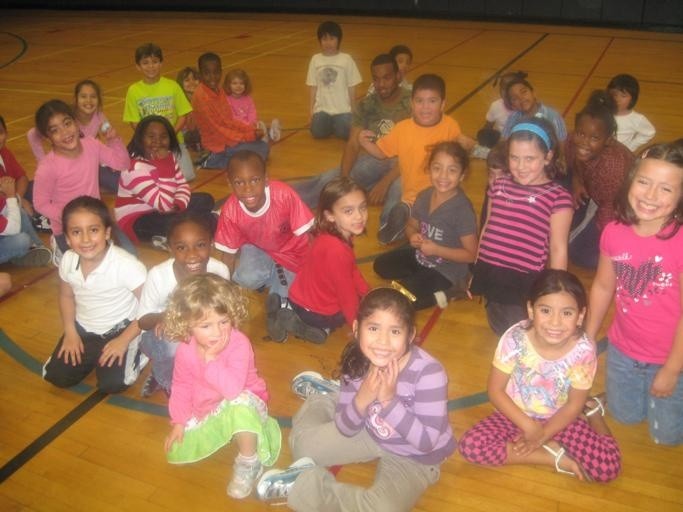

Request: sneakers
left=266, top=292, right=288, bottom=346
left=257, top=456, right=316, bottom=502
left=276, top=307, right=328, bottom=344
left=30, top=212, right=53, bottom=232
left=140, top=370, right=172, bottom=399
left=50, top=233, right=65, bottom=270
left=257, top=118, right=281, bottom=144
left=9, top=247, right=54, bottom=267
left=376, top=201, right=412, bottom=245
left=291, top=369, right=342, bottom=402
left=471, top=144, right=493, bottom=160
left=149, top=233, right=175, bottom=254
left=226, top=452, right=260, bottom=500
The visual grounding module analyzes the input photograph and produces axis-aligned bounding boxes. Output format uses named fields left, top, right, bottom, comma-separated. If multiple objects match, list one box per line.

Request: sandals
left=541, top=437, right=593, bottom=484
left=582, top=390, right=607, bottom=420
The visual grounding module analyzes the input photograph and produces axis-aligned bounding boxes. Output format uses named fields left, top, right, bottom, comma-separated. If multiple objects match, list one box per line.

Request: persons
left=1, top=21, right=683, bottom=512
